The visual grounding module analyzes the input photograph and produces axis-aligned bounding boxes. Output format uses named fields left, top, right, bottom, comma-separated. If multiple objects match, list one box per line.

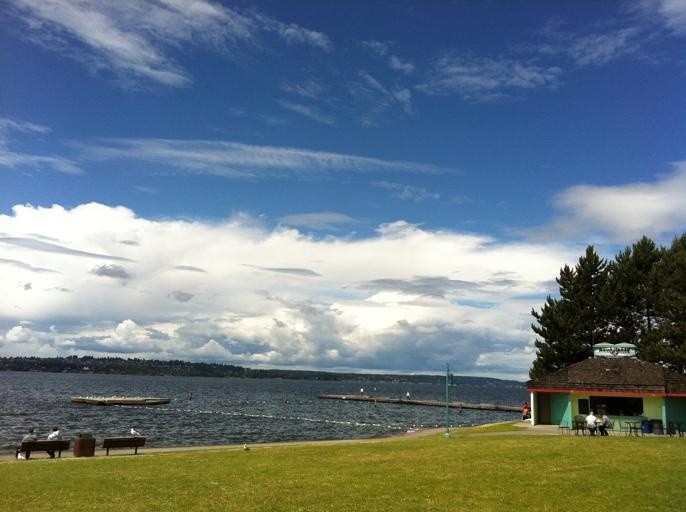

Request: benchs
left=16, top=440, right=71, bottom=460
left=103, top=437, right=146, bottom=456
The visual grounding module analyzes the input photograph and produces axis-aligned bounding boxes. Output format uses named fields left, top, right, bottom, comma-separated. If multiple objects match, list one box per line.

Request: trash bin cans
left=73, top=432, right=96, bottom=457
left=641, top=421, right=651, bottom=433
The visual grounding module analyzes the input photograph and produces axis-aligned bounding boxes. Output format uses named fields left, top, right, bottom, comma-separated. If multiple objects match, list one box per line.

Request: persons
left=360, top=386, right=363, bottom=396
left=586, top=411, right=597, bottom=435
left=598, top=413, right=609, bottom=436
left=523, top=401, right=529, bottom=420
left=406, top=391, right=410, bottom=400
left=46, top=426, right=61, bottom=458
left=23, top=426, right=37, bottom=459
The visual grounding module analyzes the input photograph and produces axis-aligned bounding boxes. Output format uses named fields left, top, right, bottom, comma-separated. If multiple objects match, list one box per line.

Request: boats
left=68, top=394, right=170, bottom=405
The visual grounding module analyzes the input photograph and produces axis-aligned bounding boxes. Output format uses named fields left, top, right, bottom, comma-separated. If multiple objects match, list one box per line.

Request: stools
left=559, top=427, right=570, bottom=436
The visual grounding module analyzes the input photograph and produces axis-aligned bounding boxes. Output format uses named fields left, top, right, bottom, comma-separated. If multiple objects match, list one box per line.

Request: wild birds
left=130, top=427, right=141, bottom=437
left=243, top=443, right=250, bottom=451
left=17, top=453, right=26, bottom=460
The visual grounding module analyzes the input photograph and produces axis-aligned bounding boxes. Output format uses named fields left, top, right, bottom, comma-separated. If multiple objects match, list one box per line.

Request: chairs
left=572, top=415, right=684, bottom=439
